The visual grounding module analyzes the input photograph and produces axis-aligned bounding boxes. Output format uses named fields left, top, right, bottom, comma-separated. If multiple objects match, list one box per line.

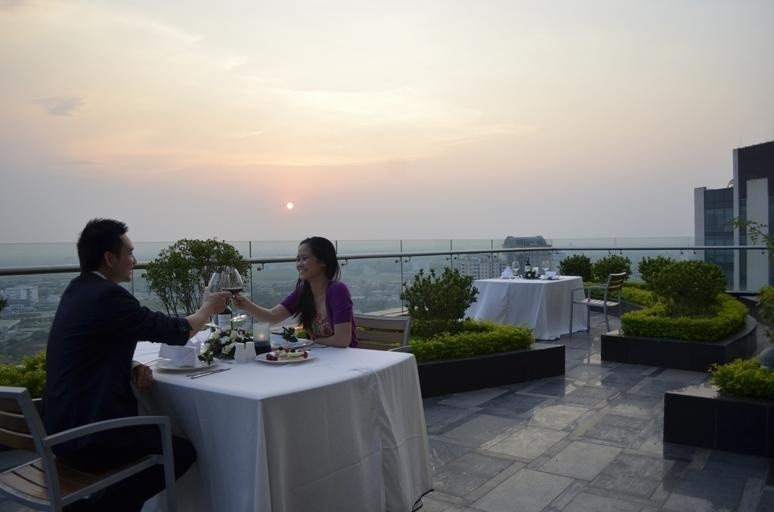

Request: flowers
left=198, top=325, right=252, bottom=365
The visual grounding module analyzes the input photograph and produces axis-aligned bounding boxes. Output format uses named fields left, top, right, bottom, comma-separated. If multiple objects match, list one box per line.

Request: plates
left=254, top=350, right=315, bottom=363
left=154, top=359, right=220, bottom=371
left=272, top=338, right=314, bottom=350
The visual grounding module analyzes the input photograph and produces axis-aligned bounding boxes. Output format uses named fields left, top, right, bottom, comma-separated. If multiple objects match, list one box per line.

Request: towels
left=544, top=271, right=556, bottom=279
left=501, top=266, right=514, bottom=278
left=159, top=340, right=202, bottom=369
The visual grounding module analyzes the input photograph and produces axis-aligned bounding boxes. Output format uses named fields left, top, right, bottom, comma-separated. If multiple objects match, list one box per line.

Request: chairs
left=0, top=387, right=182, bottom=512
left=570, top=271, right=627, bottom=337
left=353, top=314, right=411, bottom=353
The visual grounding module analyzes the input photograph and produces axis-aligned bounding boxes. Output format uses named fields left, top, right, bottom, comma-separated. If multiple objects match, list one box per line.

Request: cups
left=253, top=321, right=270, bottom=355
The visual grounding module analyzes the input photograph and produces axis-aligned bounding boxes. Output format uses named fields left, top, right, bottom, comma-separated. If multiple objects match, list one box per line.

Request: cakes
left=267, top=347, right=307, bottom=361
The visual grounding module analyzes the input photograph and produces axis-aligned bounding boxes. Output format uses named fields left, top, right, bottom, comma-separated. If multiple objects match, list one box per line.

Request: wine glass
left=203, top=272, right=230, bottom=328
left=542, top=260, right=551, bottom=278
left=221, top=267, right=248, bottom=324
left=512, top=261, right=519, bottom=276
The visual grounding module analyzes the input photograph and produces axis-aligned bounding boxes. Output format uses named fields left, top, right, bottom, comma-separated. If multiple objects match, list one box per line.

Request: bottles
left=217, top=305, right=233, bottom=329
left=525, top=257, right=531, bottom=278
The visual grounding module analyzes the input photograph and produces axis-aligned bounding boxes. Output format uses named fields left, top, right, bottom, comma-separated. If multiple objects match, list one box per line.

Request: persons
left=43, top=217, right=232, bottom=512
left=232, top=237, right=358, bottom=348
